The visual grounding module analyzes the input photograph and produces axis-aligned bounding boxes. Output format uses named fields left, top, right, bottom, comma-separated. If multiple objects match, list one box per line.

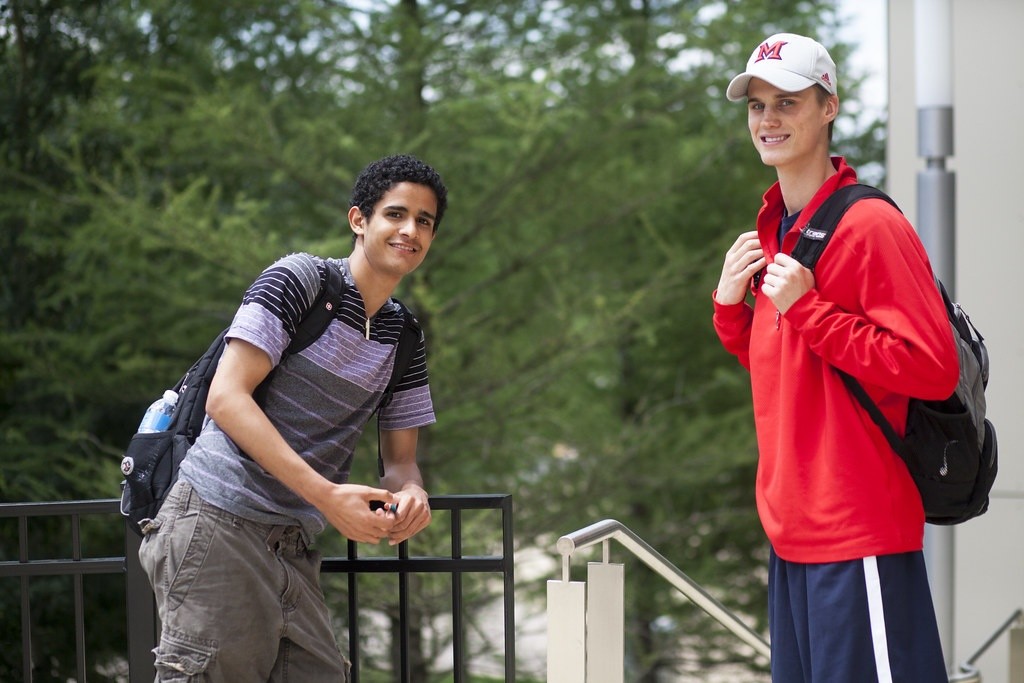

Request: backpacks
left=119, top=259, right=419, bottom=537
left=753, top=184, right=998, bottom=526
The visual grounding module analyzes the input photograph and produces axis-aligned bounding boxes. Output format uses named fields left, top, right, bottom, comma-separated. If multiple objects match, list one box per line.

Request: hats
left=727, top=32, right=837, bottom=116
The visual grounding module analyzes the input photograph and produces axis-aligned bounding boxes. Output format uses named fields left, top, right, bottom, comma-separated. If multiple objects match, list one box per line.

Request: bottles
left=136, top=390, right=179, bottom=484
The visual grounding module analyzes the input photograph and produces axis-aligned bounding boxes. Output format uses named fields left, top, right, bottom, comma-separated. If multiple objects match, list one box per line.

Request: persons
left=138, top=155, right=449, bottom=683
left=712, top=33, right=961, bottom=683
